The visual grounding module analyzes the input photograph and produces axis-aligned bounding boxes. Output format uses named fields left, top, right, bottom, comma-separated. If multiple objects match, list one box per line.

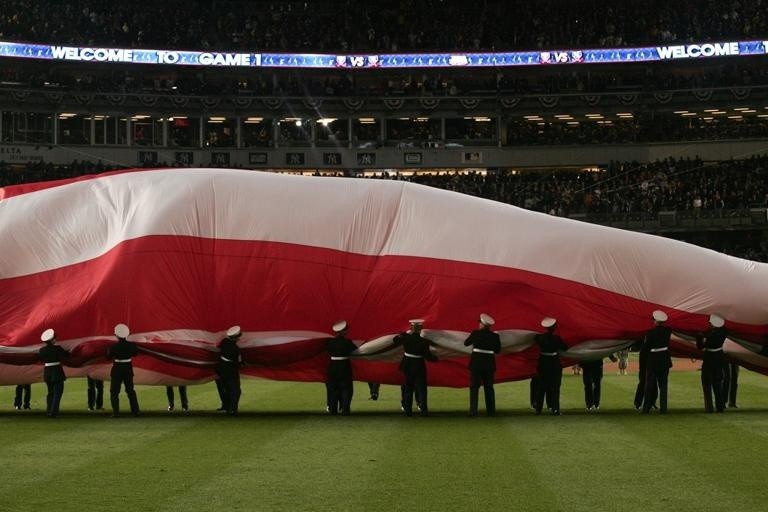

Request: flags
left=0, top=169, right=768, bottom=387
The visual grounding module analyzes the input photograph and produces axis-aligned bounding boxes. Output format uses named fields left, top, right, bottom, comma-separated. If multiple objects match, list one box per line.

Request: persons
left=636, top=308, right=675, bottom=416
left=367, top=382, right=380, bottom=401
left=665, top=231, right=768, bottom=260
left=695, top=311, right=731, bottom=414
left=167, top=386, right=189, bottom=413
left=87, top=376, right=105, bottom=413
left=214, top=379, right=229, bottom=411
left=217, top=325, right=246, bottom=417
left=326, top=320, right=358, bottom=415
left=393, top=319, right=439, bottom=415
left=463, top=312, right=503, bottom=418
left=106, top=323, right=140, bottom=418
left=13, top=384, right=32, bottom=411
left=724, top=355, right=741, bottom=410
left=39, top=329, right=69, bottom=418
left=532, top=319, right=569, bottom=415
left=582, top=357, right=603, bottom=411
left=632, top=341, right=659, bottom=411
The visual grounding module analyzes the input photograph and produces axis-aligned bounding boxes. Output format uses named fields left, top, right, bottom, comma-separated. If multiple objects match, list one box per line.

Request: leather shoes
left=650, top=405, right=658, bottom=410
left=633, top=405, right=641, bottom=411
left=221, top=410, right=228, bottom=416
left=401, top=413, right=412, bottom=418
left=703, top=408, right=714, bottom=414
left=95, top=408, right=104, bottom=411
left=584, top=406, right=594, bottom=414
left=547, top=407, right=553, bottom=414
left=417, top=411, right=430, bottom=417
left=167, top=406, right=174, bottom=413
left=88, top=408, right=94, bottom=413
left=715, top=408, right=725, bottom=414
left=368, top=396, right=373, bottom=400
left=216, top=405, right=227, bottom=411
left=371, top=394, right=378, bottom=401
left=529, top=406, right=535, bottom=410
left=324, top=410, right=338, bottom=416
left=111, top=414, right=121, bottom=419
left=593, top=404, right=600, bottom=413
left=15, top=405, right=21, bottom=411
left=231, top=411, right=239, bottom=417
left=338, top=412, right=350, bottom=418
left=46, top=411, right=56, bottom=419
left=181, top=406, right=188, bottom=413
left=132, top=412, right=141, bottom=418
left=24, top=406, right=32, bottom=411
left=416, top=404, right=423, bottom=413
left=728, top=404, right=738, bottom=408
left=399, top=405, right=406, bottom=413
left=551, top=409, right=563, bottom=416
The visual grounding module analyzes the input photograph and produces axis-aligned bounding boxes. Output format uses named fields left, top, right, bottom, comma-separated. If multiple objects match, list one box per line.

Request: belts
left=705, top=348, right=722, bottom=352
left=330, top=356, right=350, bottom=360
left=44, top=362, right=61, bottom=366
left=115, top=359, right=132, bottom=363
left=472, top=348, right=495, bottom=355
left=404, top=352, right=422, bottom=358
left=220, top=355, right=233, bottom=362
left=650, top=347, right=668, bottom=352
left=540, top=352, right=558, bottom=356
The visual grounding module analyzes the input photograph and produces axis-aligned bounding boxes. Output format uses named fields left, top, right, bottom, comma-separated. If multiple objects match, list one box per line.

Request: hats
left=114, top=324, right=129, bottom=338
left=541, top=318, right=557, bottom=328
left=480, top=313, right=495, bottom=326
left=41, top=329, right=55, bottom=342
left=227, top=326, right=241, bottom=337
left=708, top=313, right=725, bottom=328
left=332, top=320, right=346, bottom=332
left=409, top=319, right=425, bottom=326
left=652, top=310, right=668, bottom=322
